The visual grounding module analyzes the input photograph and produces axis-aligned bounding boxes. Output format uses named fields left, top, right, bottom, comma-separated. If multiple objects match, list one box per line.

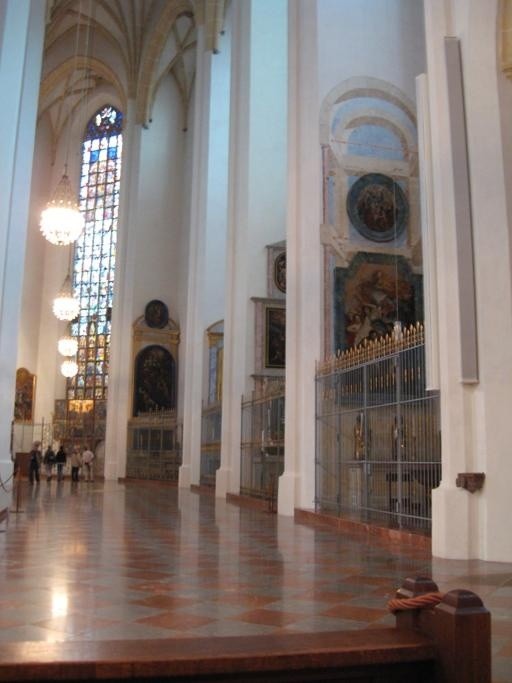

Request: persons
left=44, top=446, right=55, bottom=482
left=56, top=445, right=68, bottom=483
left=80, top=447, right=95, bottom=483
left=69, top=445, right=84, bottom=481
left=29, top=444, right=43, bottom=486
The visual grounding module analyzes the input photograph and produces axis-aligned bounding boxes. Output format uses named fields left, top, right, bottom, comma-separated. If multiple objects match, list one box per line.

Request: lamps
left=53, top=244, right=81, bottom=321
left=33, top=2, right=85, bottom=246
